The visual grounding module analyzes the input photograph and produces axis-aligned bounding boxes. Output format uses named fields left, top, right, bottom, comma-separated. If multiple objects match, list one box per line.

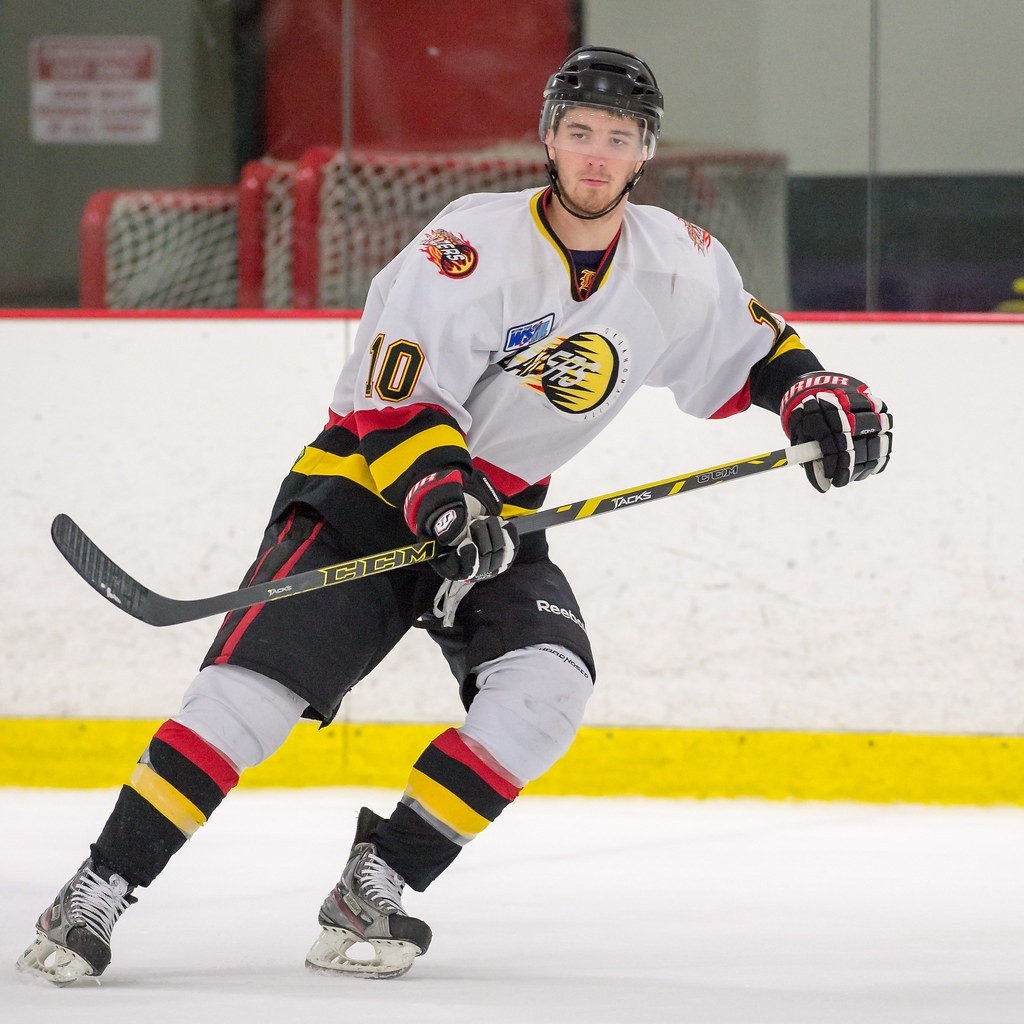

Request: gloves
left=412, top=470, right=519, bottom=583
left=780, top=372, right=893, bottom=493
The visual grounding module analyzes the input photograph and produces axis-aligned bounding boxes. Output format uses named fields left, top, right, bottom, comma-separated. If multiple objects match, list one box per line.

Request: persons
left=13, top=44, right=893, bottom=985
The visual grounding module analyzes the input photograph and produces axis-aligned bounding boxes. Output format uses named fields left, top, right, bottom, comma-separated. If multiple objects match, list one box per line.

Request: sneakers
left=16, top=855, right=139, bottom=988
left=305, top=807, right=432, bottom=980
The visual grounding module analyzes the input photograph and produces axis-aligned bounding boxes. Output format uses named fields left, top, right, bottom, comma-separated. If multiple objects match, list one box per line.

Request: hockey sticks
left=52, top=437, right=824, bottom=626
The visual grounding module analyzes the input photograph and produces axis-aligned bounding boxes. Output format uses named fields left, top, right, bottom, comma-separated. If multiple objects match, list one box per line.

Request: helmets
left=538, top=44, right=664, bottom=146
